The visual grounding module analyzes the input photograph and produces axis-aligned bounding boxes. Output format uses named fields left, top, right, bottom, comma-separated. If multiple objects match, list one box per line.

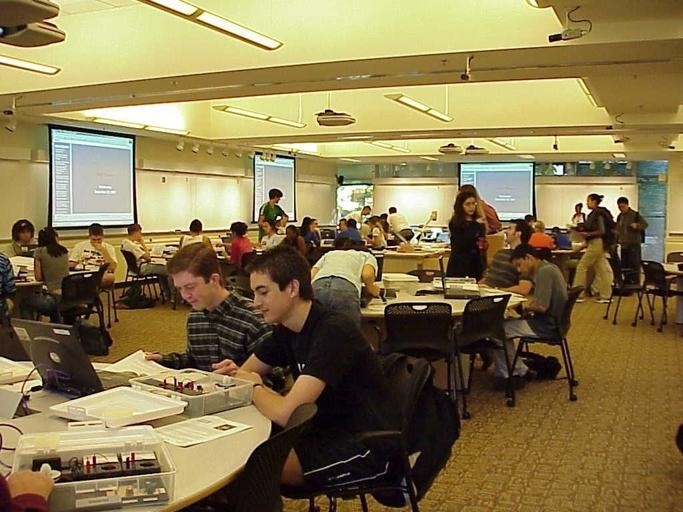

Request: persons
left=0, top=431, right=54, bottom=511
left=227, top=243, right=404, bottom=511
left=144, top=241, right=294, bottom=398
left=1, top=218, right=117, bottom=339
left=523, top=215, right=556, bottom=251
left=67, top=222, right=117, bottom=290
left=256, top=185, right=414, bottom=257
left=477, top=219, right=569, bottom=393
left=1, top=219, right=69, bottom=340
left=121, top=223, right=175, bottom=304
left=217, top=222, right=255, bottom=276
left=310, top=237, right=380, bottom=333
left=179, top=219, right=211, bottom=249
left=447, top=183, right=502, bottom=284
left=567, top=193, right=648, bottom=304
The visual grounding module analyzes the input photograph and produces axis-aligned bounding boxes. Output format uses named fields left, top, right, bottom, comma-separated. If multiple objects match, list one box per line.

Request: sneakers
left=593, top=298, right=613, bottom=303
left=575, top=298, right=585, bottom=303
left=494, top=367, right=538, bottom=391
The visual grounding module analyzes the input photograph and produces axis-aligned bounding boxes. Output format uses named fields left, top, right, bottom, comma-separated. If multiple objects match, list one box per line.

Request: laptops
left=439, top=256, right=479, bottom=298
left=9, top=318, right=136, bottom=396
left=21, top=244, right=38, bottom=257
left=150, top=244, right=165, bottom=257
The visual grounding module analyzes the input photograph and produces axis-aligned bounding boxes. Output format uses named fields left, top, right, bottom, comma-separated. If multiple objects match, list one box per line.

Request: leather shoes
left=479, top=346, right=493, bottom=369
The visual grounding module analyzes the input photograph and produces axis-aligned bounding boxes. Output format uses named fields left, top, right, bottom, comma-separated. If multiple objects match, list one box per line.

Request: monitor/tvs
left=318, top=224, right=337, bottom=238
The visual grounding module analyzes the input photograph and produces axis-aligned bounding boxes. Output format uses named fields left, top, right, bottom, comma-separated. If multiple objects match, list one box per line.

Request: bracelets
left=248, top=383, right=266, bottom=406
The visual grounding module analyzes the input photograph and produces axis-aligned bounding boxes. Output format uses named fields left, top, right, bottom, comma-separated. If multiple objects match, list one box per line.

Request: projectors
left=465, top=145, right=487, bottom=155
left=0, top=0, right=60, bottom=27
left=0, top=19, right=66, bottom=47
left=315, top=110, right=355, bottom=126
left=439, top=144, right=462, bottom=155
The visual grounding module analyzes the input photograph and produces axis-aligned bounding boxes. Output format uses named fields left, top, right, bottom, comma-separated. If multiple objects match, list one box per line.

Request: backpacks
left=369, top=352, right=461, bottom=507
left=617, top=212, right=645, bottom=243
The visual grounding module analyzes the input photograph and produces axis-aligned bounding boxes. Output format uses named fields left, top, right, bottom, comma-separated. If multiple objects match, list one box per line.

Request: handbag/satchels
left=74, top=318, right=113, bottom=356
